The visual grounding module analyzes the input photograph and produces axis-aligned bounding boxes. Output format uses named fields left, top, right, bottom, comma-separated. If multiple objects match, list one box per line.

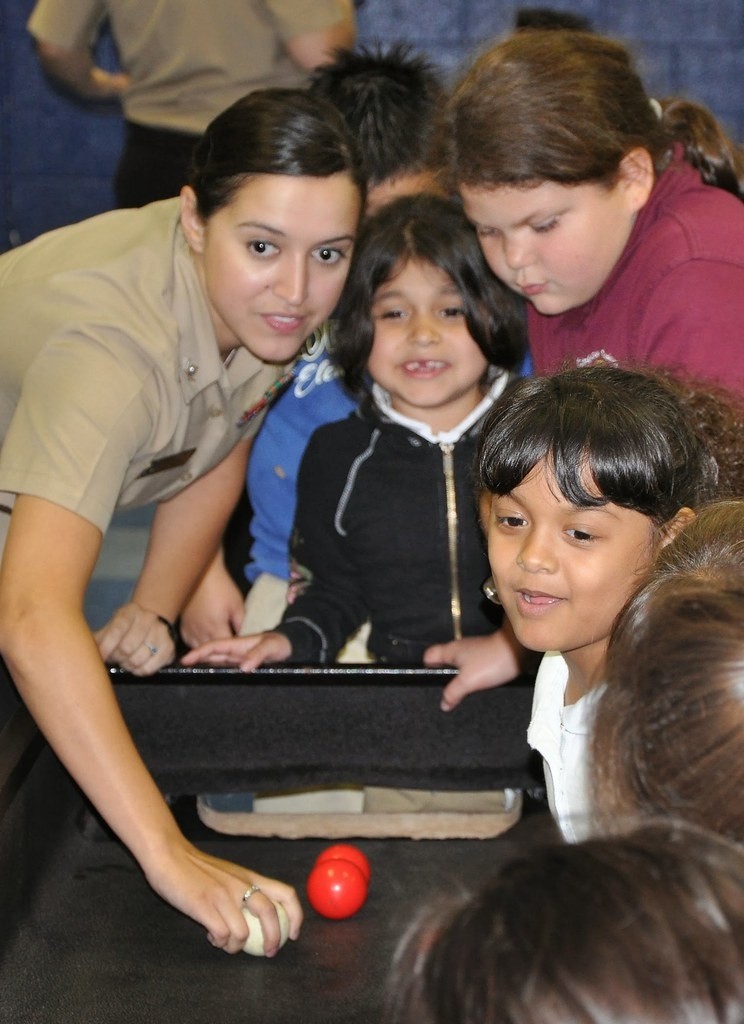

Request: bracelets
left=123, top=599, right=174, bottom=635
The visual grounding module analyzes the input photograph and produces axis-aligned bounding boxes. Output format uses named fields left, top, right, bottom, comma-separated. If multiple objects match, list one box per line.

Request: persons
left=25, top=0, right=363, bottom=213
left=381, top=365, right=744, bottom=1024
left=0, top=86, right=369, bottom=960
left=183, top=31, right=744, bottom=708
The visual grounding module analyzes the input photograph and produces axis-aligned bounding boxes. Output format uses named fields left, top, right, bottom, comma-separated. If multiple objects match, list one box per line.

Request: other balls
left=316, top=844, right=371, bottom=884
left=306, top=859, right=367, bottom=920
left=241, top=900, right=290, bottom=955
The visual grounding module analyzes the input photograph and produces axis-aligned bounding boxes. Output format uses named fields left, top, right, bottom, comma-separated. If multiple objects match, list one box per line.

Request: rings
left=243, top=886, right=261, bottom=901
left=144, top=640, right=159, bottom=655
